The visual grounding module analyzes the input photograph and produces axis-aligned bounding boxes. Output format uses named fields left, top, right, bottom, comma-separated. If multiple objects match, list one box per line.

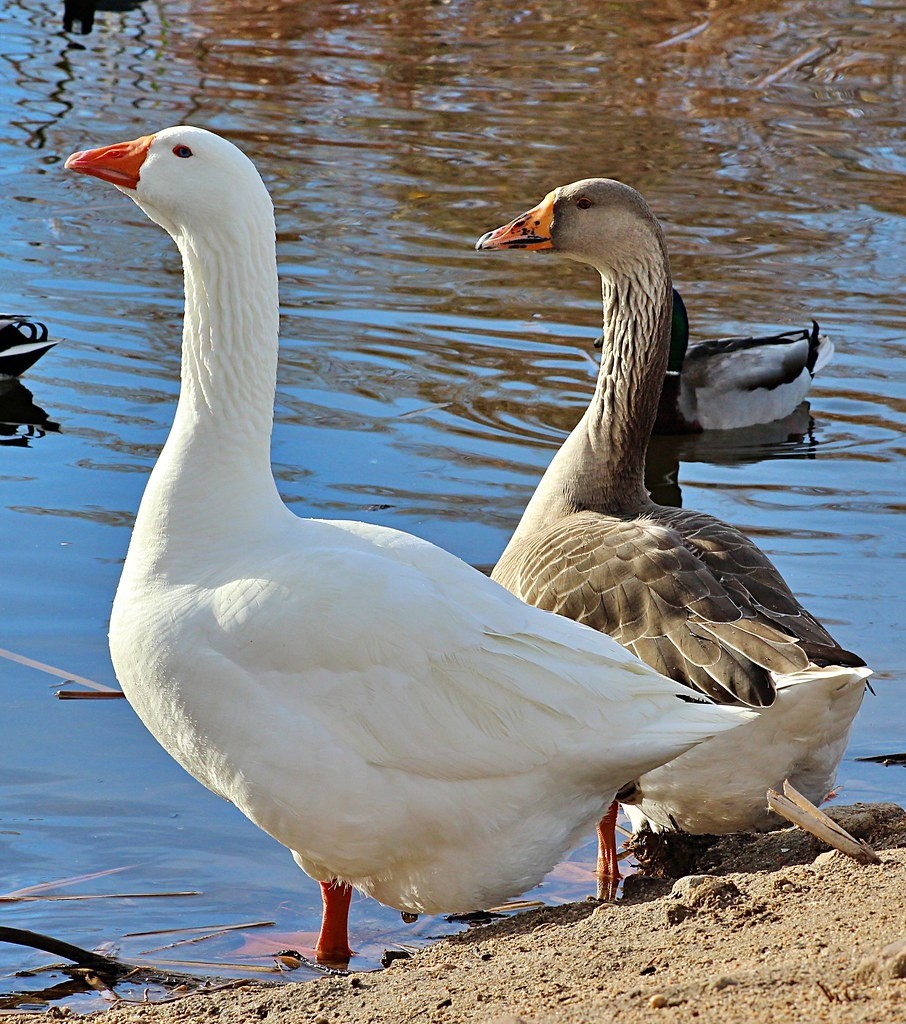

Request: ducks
left=596, top=281, right=836, bottom=432
left=63, top=124, right=760, bottom=965
left=473, top=181, right=880, bottom=903
